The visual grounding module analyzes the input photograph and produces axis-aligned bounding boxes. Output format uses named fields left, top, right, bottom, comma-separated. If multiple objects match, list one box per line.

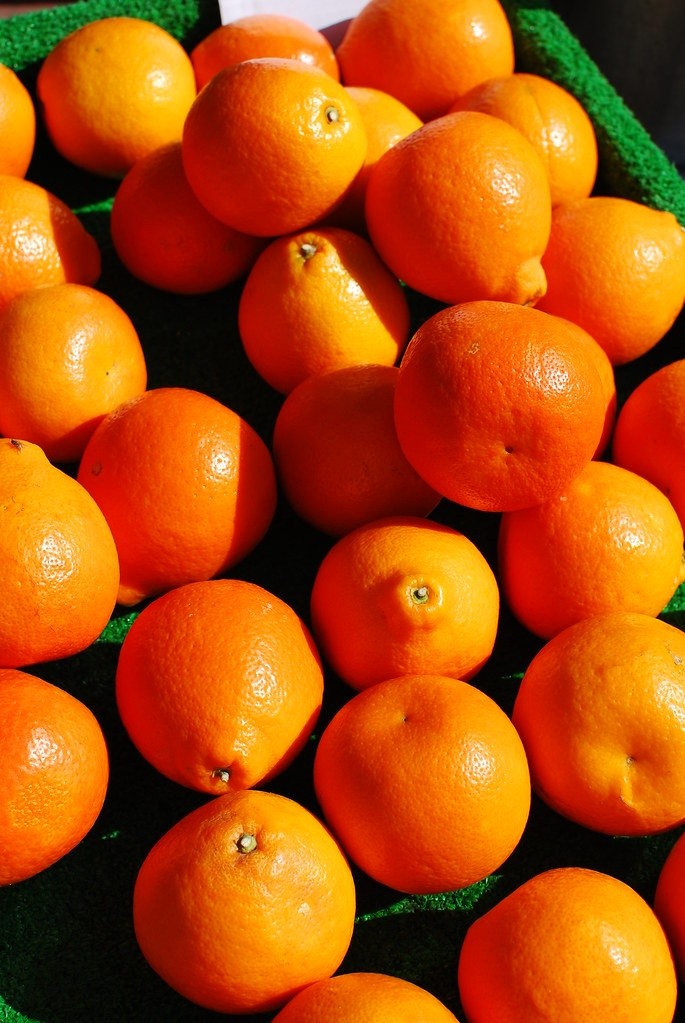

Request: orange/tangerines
left=0, top=0, right=685, bottom=1023
